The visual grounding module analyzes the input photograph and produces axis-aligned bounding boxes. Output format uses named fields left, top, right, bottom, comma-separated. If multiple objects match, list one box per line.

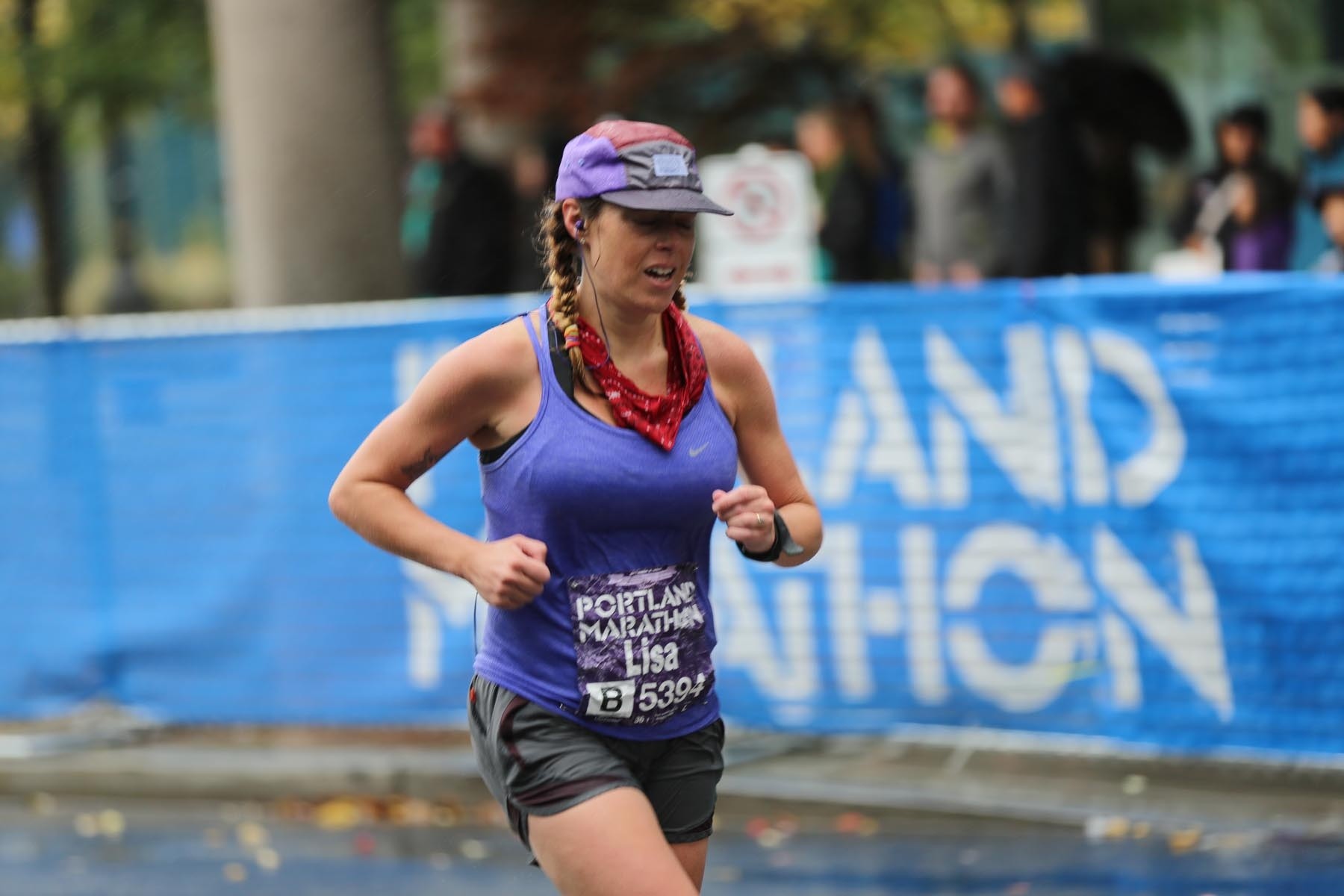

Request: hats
left=554, top=119, right=734, bottom=216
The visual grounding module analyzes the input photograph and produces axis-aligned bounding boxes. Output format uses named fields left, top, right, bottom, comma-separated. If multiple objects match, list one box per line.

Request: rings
left=757, top=514, right=763, bottom=528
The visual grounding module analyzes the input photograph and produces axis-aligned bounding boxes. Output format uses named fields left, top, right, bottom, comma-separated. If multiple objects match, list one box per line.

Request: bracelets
left=737, top=512, right=805, bottom=562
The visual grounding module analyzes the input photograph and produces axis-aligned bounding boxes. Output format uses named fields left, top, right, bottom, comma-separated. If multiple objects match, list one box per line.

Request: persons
left=391, top=45, right=1344, bottom=296
left=327, top=115, right=823, bottom=896
